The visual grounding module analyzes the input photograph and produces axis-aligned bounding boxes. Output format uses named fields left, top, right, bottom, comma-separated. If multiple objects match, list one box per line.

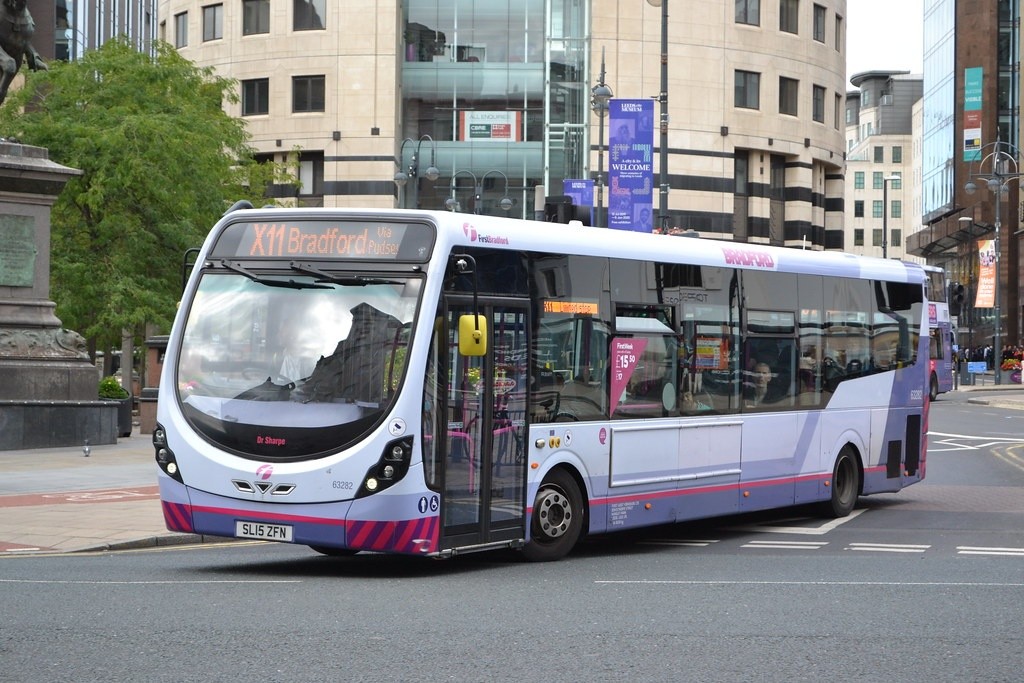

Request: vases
left=1000, top=370, right=1018, bottom=384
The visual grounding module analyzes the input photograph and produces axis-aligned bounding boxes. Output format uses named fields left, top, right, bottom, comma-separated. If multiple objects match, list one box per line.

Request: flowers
left=1001, top=359, right=1022, bottom=371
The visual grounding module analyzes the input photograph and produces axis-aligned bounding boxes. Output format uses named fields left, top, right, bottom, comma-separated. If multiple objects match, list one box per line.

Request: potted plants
left=98, top=375, right=132, bottom=437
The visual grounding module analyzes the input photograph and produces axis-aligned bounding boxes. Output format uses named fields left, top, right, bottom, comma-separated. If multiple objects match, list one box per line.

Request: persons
left=954, top=343, right=1024, bottom=374
left=652, top=346, right=815, bottom=412
left=271, top=331, right=341, bottom=402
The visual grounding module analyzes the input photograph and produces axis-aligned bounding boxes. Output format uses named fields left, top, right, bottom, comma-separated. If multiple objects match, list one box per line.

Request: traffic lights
left=949, top=283, right=965, bottom=316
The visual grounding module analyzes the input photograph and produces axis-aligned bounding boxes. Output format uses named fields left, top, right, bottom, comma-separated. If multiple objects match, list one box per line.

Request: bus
left=152, top=200, right=952, bottom=562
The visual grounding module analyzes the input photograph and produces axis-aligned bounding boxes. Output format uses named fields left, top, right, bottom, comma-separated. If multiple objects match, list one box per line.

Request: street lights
left=446, top=169, right=513, bottom=215
left=645, top=0, right=671, bottom=232
left=881, top=175, right=901, bottom=258
left=964, top=126, right=1024, bottom=385
left=393, top=134, right=440, bottom=209
left=588, top=45, right=614, bottom=228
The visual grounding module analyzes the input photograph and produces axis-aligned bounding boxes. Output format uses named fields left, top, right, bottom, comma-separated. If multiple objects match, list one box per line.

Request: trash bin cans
left=960, top=362, right=976, bottom=386
